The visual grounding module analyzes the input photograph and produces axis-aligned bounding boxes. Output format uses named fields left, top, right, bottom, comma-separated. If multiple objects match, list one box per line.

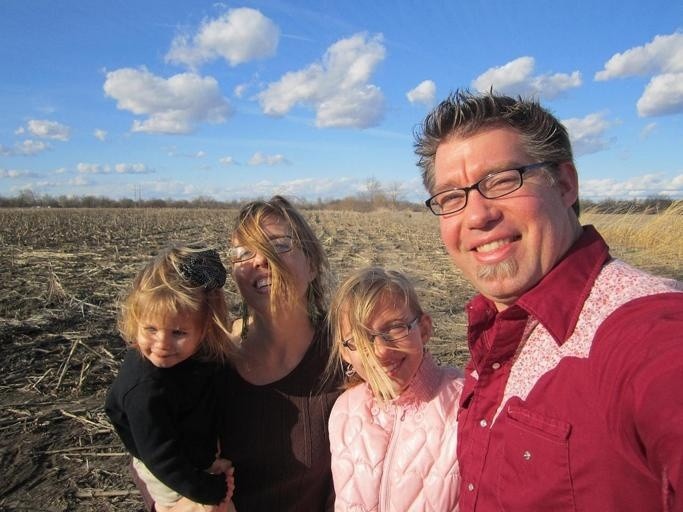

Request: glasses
left=341, top=317, right=419, bottom=352
left=230, top=235, right=294, bottom=263
left=424, top=160, right=550, bottom=216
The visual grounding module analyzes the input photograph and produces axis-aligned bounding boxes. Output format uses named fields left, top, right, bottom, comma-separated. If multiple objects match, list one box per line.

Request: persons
left=150, top=195, right=349, bottom=512
left=413, top=82, right=683, bottom=512
left=105, top=238, right=239, bottom=512
left=325, top=268, right=466, bottom=511
